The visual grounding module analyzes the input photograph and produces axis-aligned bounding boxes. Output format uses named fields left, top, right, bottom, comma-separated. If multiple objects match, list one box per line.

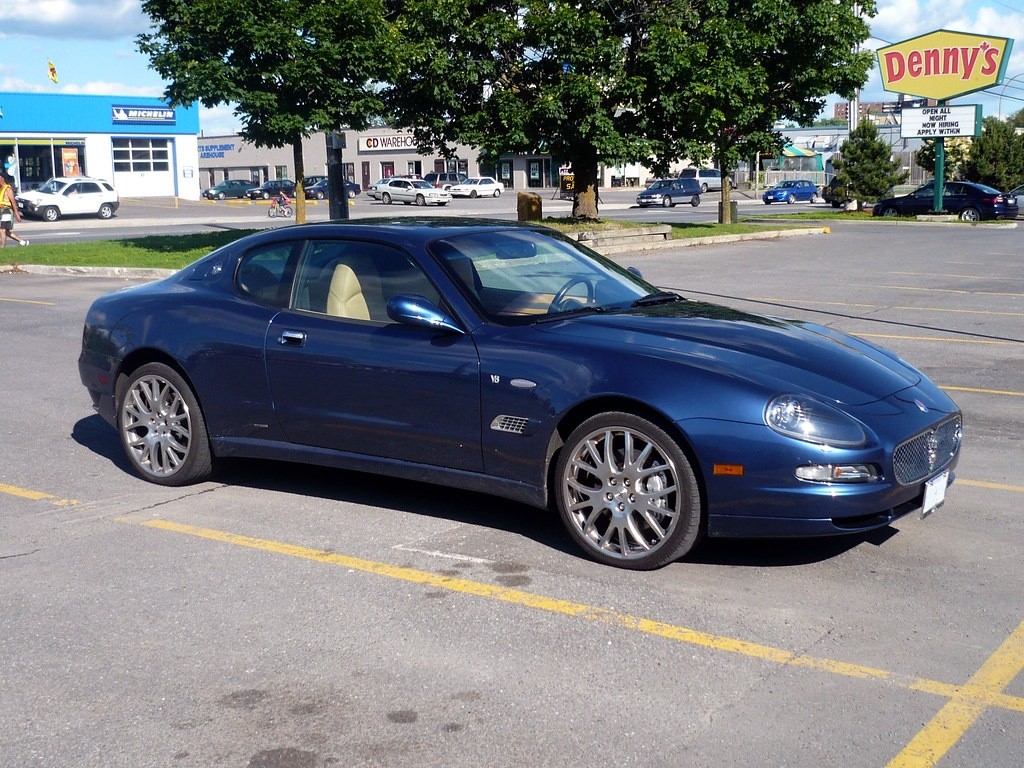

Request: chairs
left=325, top=245, right=392, bottom=324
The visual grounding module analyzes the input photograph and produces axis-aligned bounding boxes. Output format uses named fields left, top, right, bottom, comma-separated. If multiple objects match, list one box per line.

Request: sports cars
left=78, top=215, right=965, bottom=571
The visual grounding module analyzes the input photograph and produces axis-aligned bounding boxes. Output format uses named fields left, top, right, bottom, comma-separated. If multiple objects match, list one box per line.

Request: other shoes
left=24, top=240, right=29, bottom=246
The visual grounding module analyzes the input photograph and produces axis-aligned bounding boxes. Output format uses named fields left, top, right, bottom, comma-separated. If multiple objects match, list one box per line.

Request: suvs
left=636, top=179, right=703, bottom=208
left=424, top=171, right=468, bottom=190
left=820, top=175, right=895, bottom=208
left=677, top=168, right=735, bottom=193
left=14, top=176, right=120, bottom=222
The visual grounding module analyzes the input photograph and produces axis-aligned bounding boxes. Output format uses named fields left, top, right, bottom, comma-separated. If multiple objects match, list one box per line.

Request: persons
left=275, top=189, right=290, bottom=215
left=0, top=170, right=29, bottom=248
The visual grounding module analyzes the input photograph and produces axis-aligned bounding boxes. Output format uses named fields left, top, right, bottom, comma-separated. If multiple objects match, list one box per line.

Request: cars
left=1007, top=184, right=1024, bottom=215
left=449, top=177, right=505, bottom=198
left=304, top=175, right=329, bottom=187
left=202, top=179, right=258, bottom=201
left=375, top=178, right=453, bottom=206
left=305, top=179, right=361, bottom=200
left=246, top=180, right=295, bottom=200
left=366, top=174, right=423, bottom=201
left=872, top=181, right=1019, bottom=222
left=762, top=179, right=819, bottom=205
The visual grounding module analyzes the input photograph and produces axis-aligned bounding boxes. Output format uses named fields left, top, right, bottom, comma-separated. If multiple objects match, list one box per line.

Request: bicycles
left=268, top=197, right=293, bottom=218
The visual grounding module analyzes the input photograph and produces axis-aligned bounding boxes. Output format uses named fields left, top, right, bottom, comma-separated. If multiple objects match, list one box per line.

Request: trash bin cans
left=718, top=202, right=738, bottom=224
left=517, top=192, right=542, bottom=220
